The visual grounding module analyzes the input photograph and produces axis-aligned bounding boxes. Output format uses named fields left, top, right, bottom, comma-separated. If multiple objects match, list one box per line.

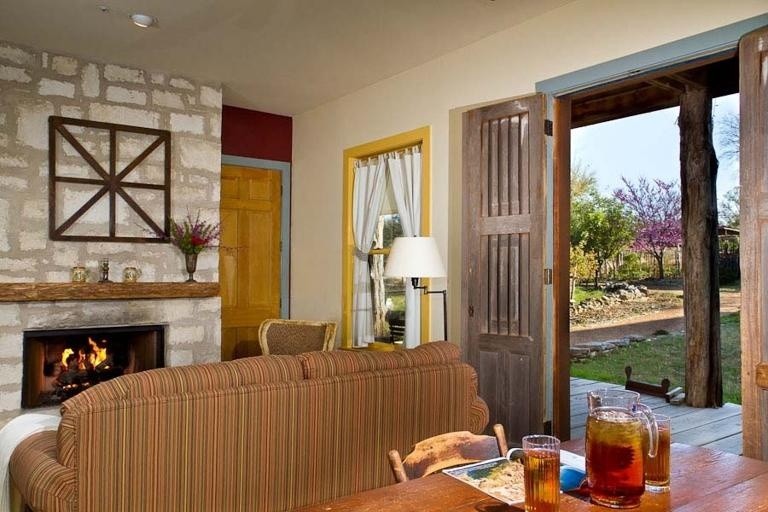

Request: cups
left=585, top=385, right=660, bottom=510
left=506, top=434, right=562, bottom=512
left=633, top=412, right=672, bottom=494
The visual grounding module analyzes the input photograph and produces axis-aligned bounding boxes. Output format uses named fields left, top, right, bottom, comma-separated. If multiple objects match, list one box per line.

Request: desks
left=296, top=438, right=767, bottom=512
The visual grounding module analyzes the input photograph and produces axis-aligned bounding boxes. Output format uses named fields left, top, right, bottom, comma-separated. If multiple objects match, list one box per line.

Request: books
left=441, top=449, right=588, bottom=507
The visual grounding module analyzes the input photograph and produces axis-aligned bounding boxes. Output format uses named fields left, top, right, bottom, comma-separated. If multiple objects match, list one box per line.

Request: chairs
left=235, top=319, right=338, bottom=357
left=387, top=421, right=506, bottom=483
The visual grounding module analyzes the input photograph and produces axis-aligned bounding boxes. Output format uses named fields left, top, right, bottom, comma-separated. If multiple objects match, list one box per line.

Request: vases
left=185, top=252, right=198, bottom=283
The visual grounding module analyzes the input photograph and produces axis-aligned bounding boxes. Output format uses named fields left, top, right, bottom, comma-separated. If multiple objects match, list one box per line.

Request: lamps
left=382, top=236, right=450, bottom=341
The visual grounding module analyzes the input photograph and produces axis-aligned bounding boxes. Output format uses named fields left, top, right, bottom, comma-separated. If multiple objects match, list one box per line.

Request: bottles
left=122, top=266, right=137, bottom=283
left=72, top=265, right=87, bottom=283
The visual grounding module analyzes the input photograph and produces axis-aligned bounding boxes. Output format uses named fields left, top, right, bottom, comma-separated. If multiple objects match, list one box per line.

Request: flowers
left=132, top=204, right=247, bottom=253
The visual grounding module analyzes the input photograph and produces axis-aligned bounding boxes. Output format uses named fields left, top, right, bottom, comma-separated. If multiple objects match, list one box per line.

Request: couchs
left=1, top=339, right=491, bottom=510
